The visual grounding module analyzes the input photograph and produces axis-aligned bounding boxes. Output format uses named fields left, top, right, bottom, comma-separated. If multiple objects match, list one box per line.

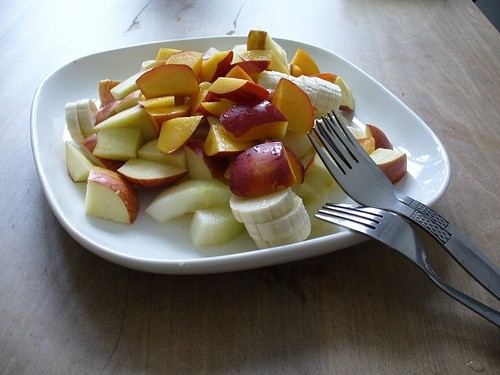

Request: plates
left=30, top=34, right=451, bottom=276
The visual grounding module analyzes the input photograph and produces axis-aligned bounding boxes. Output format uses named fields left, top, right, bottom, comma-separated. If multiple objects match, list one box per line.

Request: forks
left=316, top=109, right=500, bottom=276
left=314, top=202, right=500, bottom=327
left=306, top=117, right=500, bottom=300
